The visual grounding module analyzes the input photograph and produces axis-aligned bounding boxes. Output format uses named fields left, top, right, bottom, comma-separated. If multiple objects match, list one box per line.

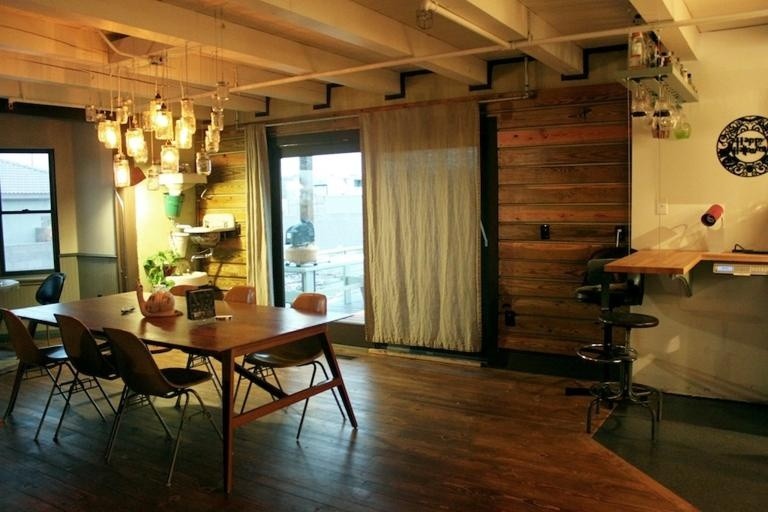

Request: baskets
left=190, top=232, right=220, bottom=247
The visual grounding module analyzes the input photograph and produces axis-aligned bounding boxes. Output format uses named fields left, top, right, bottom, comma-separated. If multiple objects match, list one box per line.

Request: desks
left=10, top=292, right=356, bottom=492
left=2, top=279, right=19, bottom=351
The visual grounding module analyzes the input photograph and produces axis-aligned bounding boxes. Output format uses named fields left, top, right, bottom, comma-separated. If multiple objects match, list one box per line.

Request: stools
left=585, top=312, right=662, bottom=441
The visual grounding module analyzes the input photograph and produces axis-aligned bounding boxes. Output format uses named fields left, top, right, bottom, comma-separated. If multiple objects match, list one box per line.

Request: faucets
left=204, top=220, right=209, bottom=228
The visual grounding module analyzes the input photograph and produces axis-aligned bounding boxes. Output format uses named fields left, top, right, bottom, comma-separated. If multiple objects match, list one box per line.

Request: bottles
left=630, top=31, right=698, bottom=93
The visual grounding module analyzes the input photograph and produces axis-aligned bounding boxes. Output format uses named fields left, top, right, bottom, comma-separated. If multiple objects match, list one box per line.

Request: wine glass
left=631, top=82, right=691, bottom=140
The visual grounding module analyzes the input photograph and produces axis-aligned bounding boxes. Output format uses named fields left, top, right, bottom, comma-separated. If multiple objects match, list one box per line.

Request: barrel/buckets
left=170, top=232, right=189, bottom=258
left=163, top=192, right=184, bottom=218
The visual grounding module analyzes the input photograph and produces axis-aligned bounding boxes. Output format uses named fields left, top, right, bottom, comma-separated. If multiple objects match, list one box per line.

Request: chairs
left=101, top=327, right=221, bottom=489
left=169, top=285, right=200, bottom=295
left=2, top=308, right=109, bottom=425
left=564, top=248, right=645, bottom=396
left=234, top=293, right=349, bottom=439
left=52, top=314, right=174, bottom=441
left=205, top=286, right=275, bottom=405
left=33, top=273, right=65, bottom=347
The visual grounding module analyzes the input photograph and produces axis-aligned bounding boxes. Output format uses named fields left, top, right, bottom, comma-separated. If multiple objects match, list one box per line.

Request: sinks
left=166, top=271, right=209, bottom=286
left=185, top=226, right=225, bottom=247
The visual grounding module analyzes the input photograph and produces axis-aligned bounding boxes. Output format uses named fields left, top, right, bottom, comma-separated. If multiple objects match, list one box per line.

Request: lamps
left=701, top=204, right=724, bottom=227
left=83, top=6, right=231, bottom=198
